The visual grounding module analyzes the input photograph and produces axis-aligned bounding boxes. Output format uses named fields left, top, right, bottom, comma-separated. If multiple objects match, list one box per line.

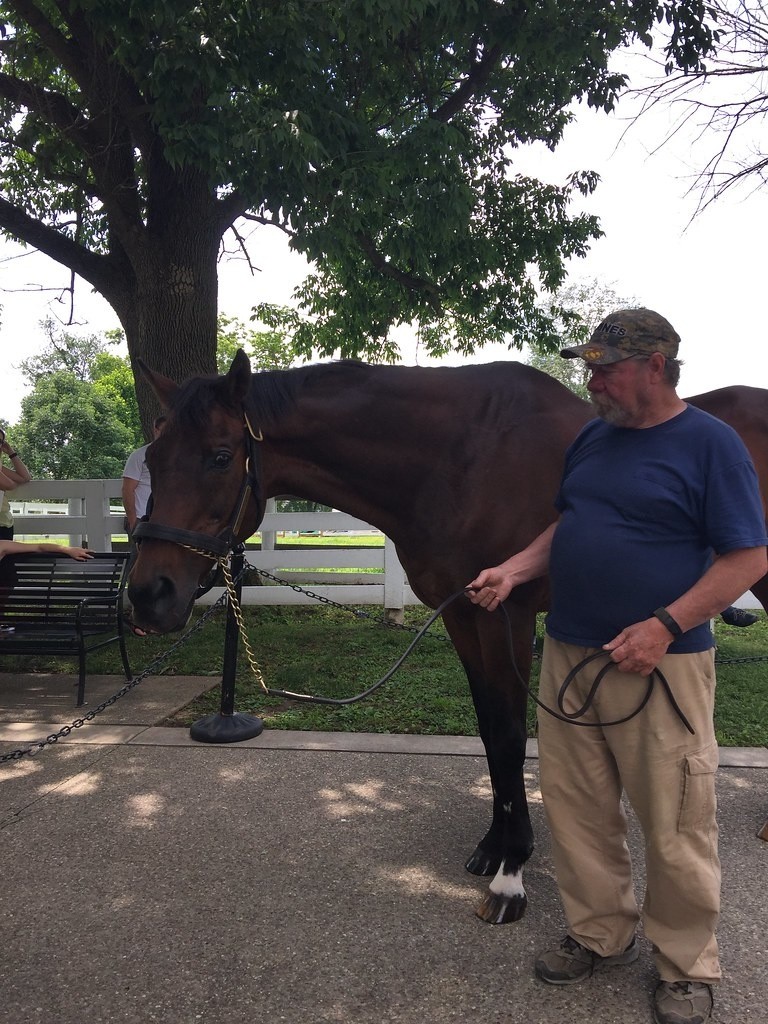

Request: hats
left=560, top=308, right=680, bottom=365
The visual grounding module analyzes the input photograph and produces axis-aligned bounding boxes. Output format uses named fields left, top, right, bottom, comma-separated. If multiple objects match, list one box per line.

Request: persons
left=121, top=416, right=172, bottom=636
left=462, top=307, right=766, bottom=1024
left=0, top=428, right=96, bottom=632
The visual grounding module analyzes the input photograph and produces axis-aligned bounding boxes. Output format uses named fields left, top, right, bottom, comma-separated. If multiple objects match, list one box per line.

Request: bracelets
left=9, top=452, right=17, bottom=458
left=653, top=607, right=684, bottom=640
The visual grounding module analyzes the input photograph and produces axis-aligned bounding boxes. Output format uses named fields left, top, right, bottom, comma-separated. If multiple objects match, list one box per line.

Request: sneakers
left=653, top=980, right=714, bottom=1024
left=535, top=934, right=640, bottom=985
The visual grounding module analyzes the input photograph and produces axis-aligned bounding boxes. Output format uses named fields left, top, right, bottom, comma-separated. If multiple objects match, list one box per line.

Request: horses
left=126, top=348, right=768, bottom=927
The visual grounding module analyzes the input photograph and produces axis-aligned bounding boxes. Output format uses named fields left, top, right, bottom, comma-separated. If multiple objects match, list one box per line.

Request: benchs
left=0, top=551, right=133, bottom=709
